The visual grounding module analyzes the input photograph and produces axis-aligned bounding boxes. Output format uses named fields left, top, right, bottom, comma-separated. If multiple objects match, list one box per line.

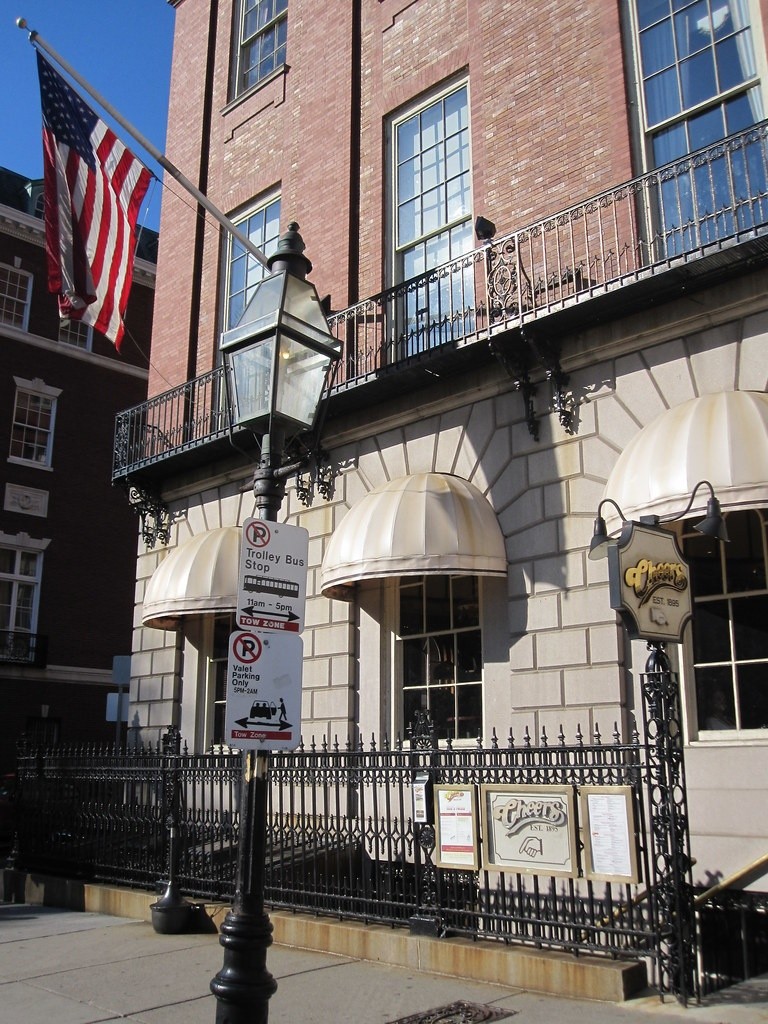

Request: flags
left=30, top=44, right=158, bottom=354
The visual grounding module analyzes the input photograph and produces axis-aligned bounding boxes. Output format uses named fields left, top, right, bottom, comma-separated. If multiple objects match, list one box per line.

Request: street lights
left=211, top=222, right=347, bottom=1023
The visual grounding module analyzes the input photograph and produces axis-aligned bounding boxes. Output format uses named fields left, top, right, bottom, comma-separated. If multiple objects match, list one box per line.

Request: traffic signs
left=237, top=514, right=307, bottom=639
left=224, top=627, right=303, bottom=753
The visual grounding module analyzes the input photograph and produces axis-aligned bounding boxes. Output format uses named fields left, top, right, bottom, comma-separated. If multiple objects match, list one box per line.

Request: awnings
left=595, top=390, right=767, bottom=552
left=140, top=526, right=245, bottom=632
left=318, top=471, right=508, bottom=601
